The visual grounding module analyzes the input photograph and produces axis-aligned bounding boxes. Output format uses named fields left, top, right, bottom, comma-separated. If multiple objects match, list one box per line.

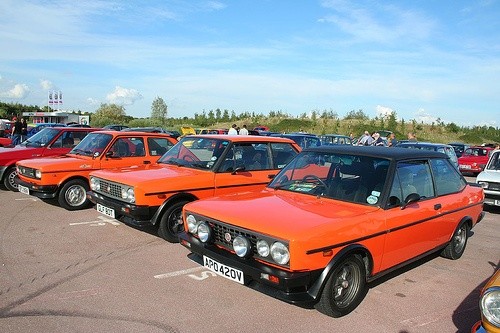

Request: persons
left=228, top=124, right=237, bottom=135
left=251, top=128, right=259, bottom=135
left=489, top=143, right=500, bottom=157
left=388, top=133, right=396, bottom=146
left=349, top=131, right=384, bottom=146
left=408, top=132, right=417, bottom=142
left=299, top=129, right=304, bottom=133
left=11, top=117, right=27, bottom=145
left=239, top=125, right=248, bottom=135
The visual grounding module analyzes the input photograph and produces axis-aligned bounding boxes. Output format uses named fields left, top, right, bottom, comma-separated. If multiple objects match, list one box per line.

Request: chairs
left=133, top=144, right=144, bottom=155
left=242, top=144, right=261, bottom=170
left=338, top=164, right=360, bottom=184
left=63, top=137, right=74, bottom=146
left=114, top=142, right=130, bottom=156
left=391, top=168, right=417, bottom=201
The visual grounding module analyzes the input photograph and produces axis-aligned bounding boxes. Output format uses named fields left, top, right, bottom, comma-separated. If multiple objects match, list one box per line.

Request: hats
left=12, top=117, right=17, bottom=120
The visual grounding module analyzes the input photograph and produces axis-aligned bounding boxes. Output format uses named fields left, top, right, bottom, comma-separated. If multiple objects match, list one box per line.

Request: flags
left=48, top=91, right=63, bottom=105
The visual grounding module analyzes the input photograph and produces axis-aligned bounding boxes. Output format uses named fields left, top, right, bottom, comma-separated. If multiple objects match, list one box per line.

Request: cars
left=68, top=124, right=92, bottom=141
left=86, top=135, right=336, bottom=243
left=13, top=130, right=201, bottom=211
left=447, top=142, right=471, bottom=158
left=177, top=144, right=486, bottom=319
left=101, top=125, right=409, bottom=166
left=0, top=126, right=137, bottom=192
left=475, top=149, right=500, bottom=209
left=374, top=142, right=459, bottom=179
left=0, top=119, right=74, bottom=148
left=457, top=146, right=496, bottom=176
